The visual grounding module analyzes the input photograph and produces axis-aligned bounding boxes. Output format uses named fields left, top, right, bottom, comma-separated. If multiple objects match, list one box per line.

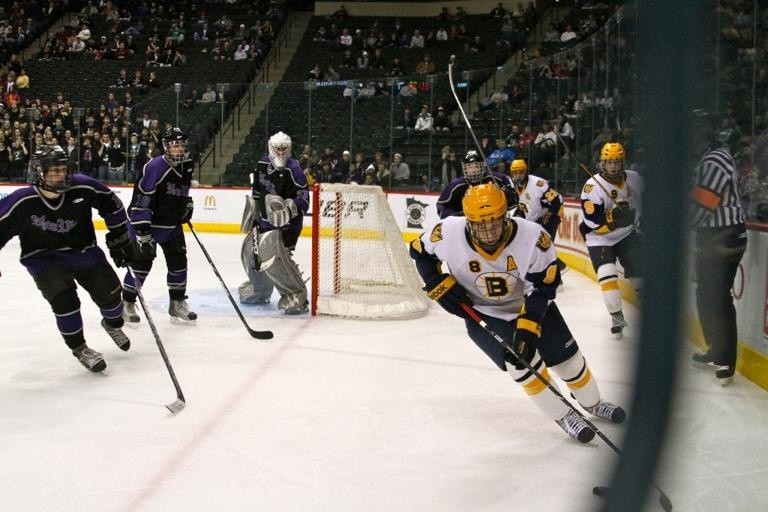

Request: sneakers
left=693, top=349, right=713, bottom=363
left=716, top=363, right=735, bottom=378
left=591, top=400, right=625, bottom=424
left=556, top=409, right=594, bottom=443
left=611, top=312, right=624, bottom=333
left=73, top=298, right=196, bottom=373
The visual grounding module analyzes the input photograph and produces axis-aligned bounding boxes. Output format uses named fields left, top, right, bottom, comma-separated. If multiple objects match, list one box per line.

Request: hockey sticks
left=553, top=125, right=645, bottom=239
left=250, top=172, right=275, bottom=273
left=454, top=294, right=673, bottom=511
left=447, top=54, right=500, bottom=189
left=124, top=263, right=186, bottom=417
left=187, top=219, right=273, bottom=339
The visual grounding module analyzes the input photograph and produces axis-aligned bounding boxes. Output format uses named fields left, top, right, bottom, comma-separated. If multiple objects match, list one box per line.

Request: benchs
left=0, top=0, right=602, bottom=196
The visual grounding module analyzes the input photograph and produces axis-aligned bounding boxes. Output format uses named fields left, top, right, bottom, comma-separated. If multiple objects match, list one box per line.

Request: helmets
left=163, top=125, right=189, bottom=163
left=267, top=132, right=292, bottom=172
left=28, top=145, right=74, bottom=194
left=461, top=141, right=627, bottom=250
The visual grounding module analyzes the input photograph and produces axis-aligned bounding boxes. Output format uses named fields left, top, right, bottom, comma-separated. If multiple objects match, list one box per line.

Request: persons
left=507, top=159, right=568, bottom=291
left=688, top=119, right=747, bottom=385
left=123, top=127, right=198, bottom=323
left=580, top=143, right=647, bottom=342
left=239, top=130, right=311, bottom=315
left=1, top=145, right=142, bottom=373
left=408, top=181, right=627, bottom=442
left=1, top=0, right=766, bottom=223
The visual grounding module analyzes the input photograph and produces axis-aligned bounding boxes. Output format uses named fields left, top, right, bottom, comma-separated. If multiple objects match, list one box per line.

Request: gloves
left=181, top=197, right=194, bottom=224
left=136, top=225, right=155, bottom=258
left=106, top=226, right=136, bottom=268
left=423, top=273, right=473, bottom=320
left=506, top=313, right=542, bottom=371
